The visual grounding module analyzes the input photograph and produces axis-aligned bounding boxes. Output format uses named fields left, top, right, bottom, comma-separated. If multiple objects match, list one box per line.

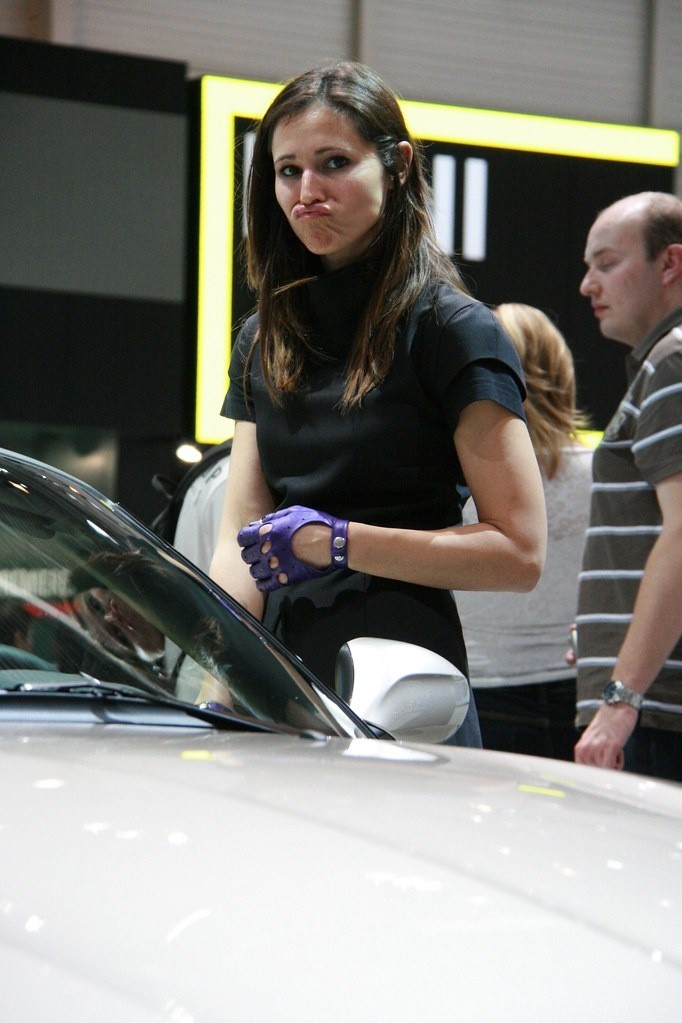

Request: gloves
left=236, top=505, right=348, bottom=592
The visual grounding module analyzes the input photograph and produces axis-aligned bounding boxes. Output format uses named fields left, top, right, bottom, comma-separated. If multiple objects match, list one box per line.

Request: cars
left=1, top=446, right=682, bottom=1022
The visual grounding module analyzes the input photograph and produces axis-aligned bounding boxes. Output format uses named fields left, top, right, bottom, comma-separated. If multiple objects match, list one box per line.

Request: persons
left=62, top=548, right=229, bottom=676
left=199, top=60, right=551, bottom=749
left=566, top=190, right=682, bottom=782
left=448, top=304, right=596, bottom=761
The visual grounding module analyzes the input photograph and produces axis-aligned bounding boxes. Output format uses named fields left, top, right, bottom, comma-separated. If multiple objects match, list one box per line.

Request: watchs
left=601, top=680, right=644, bottom=710
